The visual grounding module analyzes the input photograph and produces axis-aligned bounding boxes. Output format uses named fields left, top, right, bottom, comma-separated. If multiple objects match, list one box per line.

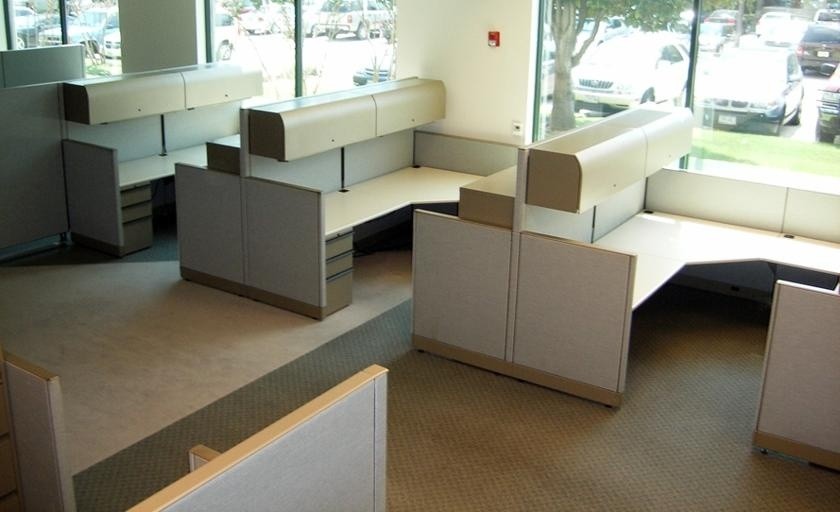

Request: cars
left=11, top=1, right=121, bottom=58
left=210, top=2, right=394, bottom=86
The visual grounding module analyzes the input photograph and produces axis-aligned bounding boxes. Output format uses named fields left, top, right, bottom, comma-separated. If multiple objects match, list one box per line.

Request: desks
left=590, top=209, right=840, bottom=312
left=323, top=165, right=487, bottom=318
left=118, top=144, right=207, bottom=257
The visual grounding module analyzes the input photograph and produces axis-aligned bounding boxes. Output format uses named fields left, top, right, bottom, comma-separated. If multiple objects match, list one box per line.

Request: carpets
left=71, top=283, right=840, bottom=512
